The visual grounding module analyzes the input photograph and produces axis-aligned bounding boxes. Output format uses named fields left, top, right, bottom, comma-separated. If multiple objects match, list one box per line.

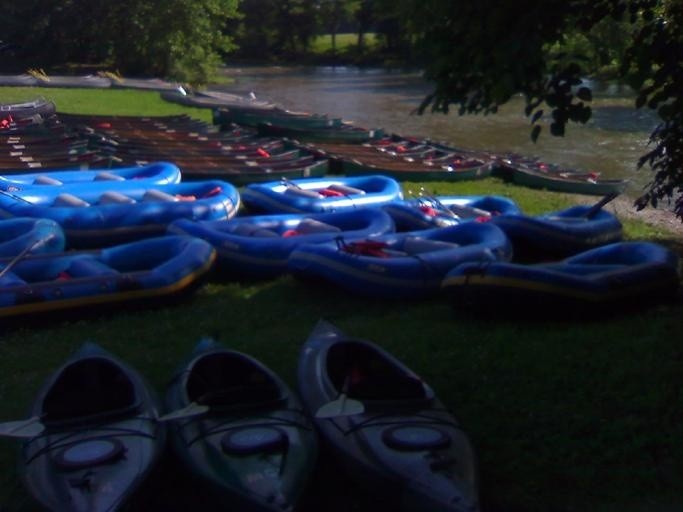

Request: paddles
left=315, top=373, right=365, bottom=417
left=0, top=414, right=44, bottom=437
left=156, top=393, right=207, bottom=421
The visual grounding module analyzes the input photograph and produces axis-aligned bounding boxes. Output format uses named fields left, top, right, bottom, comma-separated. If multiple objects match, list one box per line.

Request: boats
left=164, top=333, right=324, bottom=508
left=19, top=338, right=171, bottom=509
left=295, top=329, right=481, bottom=512
